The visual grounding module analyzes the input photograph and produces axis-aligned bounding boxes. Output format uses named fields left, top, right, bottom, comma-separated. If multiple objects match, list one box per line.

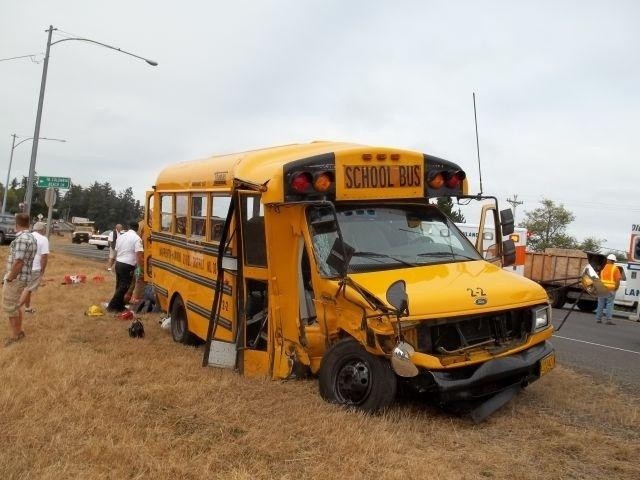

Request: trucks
left=522, top=241, right=640, bottom=323
left=418, top=219, right=528, bottom=280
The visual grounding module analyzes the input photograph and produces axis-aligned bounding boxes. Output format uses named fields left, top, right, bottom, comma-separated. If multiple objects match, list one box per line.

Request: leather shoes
left=597, top=320, right=602, bottom=323
left=606, top=320, right=616, bottom=325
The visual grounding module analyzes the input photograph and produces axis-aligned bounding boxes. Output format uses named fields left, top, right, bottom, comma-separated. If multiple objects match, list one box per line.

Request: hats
left=33, top=221, right=47, bottom=230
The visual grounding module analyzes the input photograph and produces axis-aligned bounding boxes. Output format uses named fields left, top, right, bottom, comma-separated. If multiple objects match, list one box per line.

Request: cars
left=88, top=230, right=114, bottom=250
left=51, top=219, right=65, bottom=224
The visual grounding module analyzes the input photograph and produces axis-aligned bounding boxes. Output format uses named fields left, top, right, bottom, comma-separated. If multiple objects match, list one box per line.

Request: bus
left=139, top=136, right=559, bottom=425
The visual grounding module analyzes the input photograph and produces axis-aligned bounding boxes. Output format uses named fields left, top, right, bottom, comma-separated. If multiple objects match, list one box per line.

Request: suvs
left=0, top=214, right=23, bottom=245
left=72, top=225, right=98, bottom=245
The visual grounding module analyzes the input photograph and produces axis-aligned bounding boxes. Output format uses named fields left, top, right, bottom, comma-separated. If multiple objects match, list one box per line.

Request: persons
left=105, top=211, right=153, bottom=311
left=20, top=222, right=49, bottom=312
left=1, top=213, right=38, bottom=346
left=595, top=253, right=623, bottom=325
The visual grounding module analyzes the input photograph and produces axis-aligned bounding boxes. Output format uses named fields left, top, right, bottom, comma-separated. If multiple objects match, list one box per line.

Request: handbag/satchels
left=84, top=304, right=104, bottom=317
left=129, top=319, right=145, bottom=339
left=114, top=311, right=134, bottom=320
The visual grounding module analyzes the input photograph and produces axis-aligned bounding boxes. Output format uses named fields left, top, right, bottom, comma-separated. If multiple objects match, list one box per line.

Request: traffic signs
left=36, top=175, right=71, bottom=189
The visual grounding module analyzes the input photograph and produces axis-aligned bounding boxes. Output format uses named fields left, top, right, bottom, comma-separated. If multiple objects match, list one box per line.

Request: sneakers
left=106, top=306, right=127, bottom=312
left=3, top=331, right=25, bottom=348
left=25, top=306, right=36, bottom=314
left=107, top=266, right=112, bottom=271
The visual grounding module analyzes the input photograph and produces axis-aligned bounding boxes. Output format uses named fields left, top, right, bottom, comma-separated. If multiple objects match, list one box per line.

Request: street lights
left=1, top=134, right=66, bottom=227
left=18, top=21, right=159, bottom=228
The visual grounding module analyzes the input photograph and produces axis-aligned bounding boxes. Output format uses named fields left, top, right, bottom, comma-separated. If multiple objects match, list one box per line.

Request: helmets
left=607, top=253, right=617, bottom=262
